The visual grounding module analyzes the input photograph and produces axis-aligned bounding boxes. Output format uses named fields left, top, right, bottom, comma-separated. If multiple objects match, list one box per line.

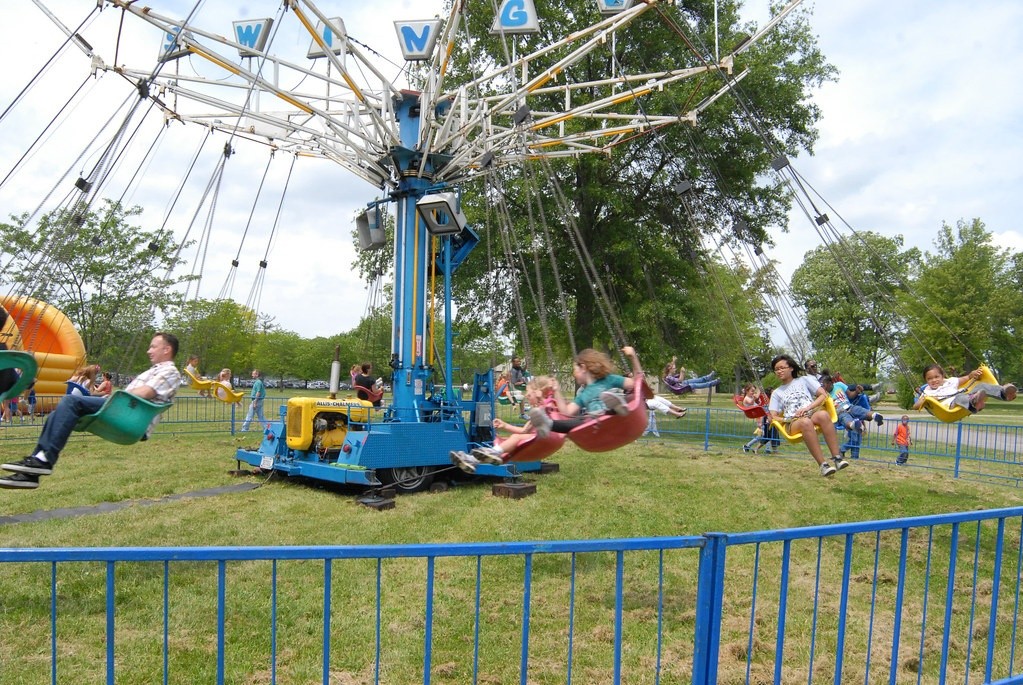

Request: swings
left=461, top=0, right=1000, bottom=463
left=0, top=0, right=391, bottom=444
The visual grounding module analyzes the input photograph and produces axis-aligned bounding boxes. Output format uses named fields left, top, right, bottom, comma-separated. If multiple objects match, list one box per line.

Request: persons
left=0, top=308, right=18, bottom=394
left=623, top=372, right=687, bottom=420
left=508, top=358, right=535, bottom=386
left=349, top=362, right=385, bottom=411
left=769, top=354, right=849, bottom=476
left=0, top=332, right=180, bottom=488
left=0, top=388, right=37, bottom=421
left=890, top=414, right=913, bottom=466
left=742, top=384, right=780, bottom=453
left=240, top=370, right=268, bottom=432
left=663, top=355, right=721, bottom=390
left=805, top=361, right=896, bottom=459
left=530, top=346, right=644, bottom=439
left=93, top=372, right=112, bottom=395
left=449, top=375, right=570, bottom=473
left=914, top=364, right=1017, bottom=414
left=495, top=371, right=531, bottom=420
left=69, top=365, right=109, bottom=396
left=187, top=356, right=213, bottom=398
left=217, top=369, right=243, bottom=407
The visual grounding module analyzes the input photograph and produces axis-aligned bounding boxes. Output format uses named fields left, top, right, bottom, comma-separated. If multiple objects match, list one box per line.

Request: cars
left=887, top=388, right=895, bottom=393
left=95, top=373, right=131, bottom=384
left=233, top=378, right=352, bottom=391
left=384, top=386, right=391, bottom=392
left=180, top=378, right=186, bottom=385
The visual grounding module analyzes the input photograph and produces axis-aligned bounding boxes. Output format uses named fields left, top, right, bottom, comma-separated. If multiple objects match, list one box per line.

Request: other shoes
left=712, top=378, right=721, bottom=386
left=773, top=450, right=785, bottom=456
left=743, top=445, right=748, bottom=453
left=675, top=412, right=685, bottom=419
left=677, top=408, right=687, bottom=412
left=839, top=448, right=845, bottom=458
left=872, top=381, right=883, bottom=393
left=1, top=455, right=53, bottom=475
left=519, top=413, right=531, bottom=420
left=471, top=447, right=504, bottom=466
left=1001, top=383, right=1018, bottom=401
left=524, top=406, right=531, bottom=411
left=449, top=450, right=476, bottom=474
left=970, top=389, right=986, bottom=414
left=599, top=392, right=630, bottom=417
left=763, top=450, right=772, bottom=456
left=868, top=392, right=880, bottom=405
left=529, top=407, right=551, bottom=439
left=710, top=370, right=715, bottom=380
left=0, top=473, right=40, bottom=489
left=895, top=459, right=898, bottom=466
left=820, top=462, right=836, bottom=476
left=752, top=448, right=758, bottom=455
left=829, top=455, right=849, bottom=471
left=873, top=413, right=884, bottom=426
left=852, top=418, right=863, bottom=434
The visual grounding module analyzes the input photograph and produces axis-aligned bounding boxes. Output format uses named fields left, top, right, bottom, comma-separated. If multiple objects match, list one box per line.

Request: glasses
left=773, top=366, right=790, bottom=372
